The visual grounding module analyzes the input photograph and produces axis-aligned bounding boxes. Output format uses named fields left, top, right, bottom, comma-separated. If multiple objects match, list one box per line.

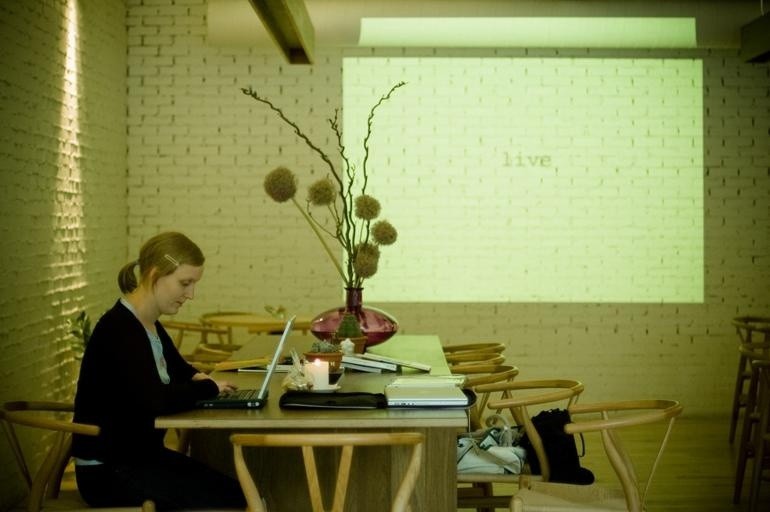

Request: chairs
left=448, top=365, right=518, bottom=427
left=222, top=431, right=424, bottom=512
left=454, top=379, right=584, bottom=505
left=727, top=316, right=768, bottom=443
left=509, top=400, right=682, bottom=511
left=1, top=399, right=155, bottom=508
left=749, top=357, right=770, bottom=511
left=732, top=342, right=768, bottom=509
left=152, top=318, right=231, bottom=361
left=445, top=351, right=506, bottom=365
left=198, top=309, right=262, bottom=355
left=442, top=340, right=507, bottom=355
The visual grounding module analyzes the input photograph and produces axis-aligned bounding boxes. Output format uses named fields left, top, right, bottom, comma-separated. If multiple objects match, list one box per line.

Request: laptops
left=384, top=373, right=469, bottom=407
left=195, top=310, right=298, bottom=410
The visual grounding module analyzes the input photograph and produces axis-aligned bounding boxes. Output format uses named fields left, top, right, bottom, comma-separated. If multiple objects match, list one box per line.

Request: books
left=340, top=363, right=382, bottom=374
left=214, top=358, right=270, bottom=371
left=257, top=362, right=304, bottom=372
left=236, top=367, right=289, bottom=373
left=343, top=354, right=399, bottom=371
left=362, top=352, right=432, bottom=373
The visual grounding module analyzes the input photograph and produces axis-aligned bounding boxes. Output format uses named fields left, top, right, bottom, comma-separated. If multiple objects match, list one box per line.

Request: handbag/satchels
left=457, top=408, right=527, bottom=479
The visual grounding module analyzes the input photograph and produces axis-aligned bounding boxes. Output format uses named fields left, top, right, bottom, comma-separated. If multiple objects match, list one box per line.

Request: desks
left=212, top=314, right=311, bottom=341
left=154, top=332, right=469, bottom=511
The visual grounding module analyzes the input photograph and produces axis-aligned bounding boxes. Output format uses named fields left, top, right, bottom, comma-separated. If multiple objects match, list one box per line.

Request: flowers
left=240, top=80, right=407, bottom=286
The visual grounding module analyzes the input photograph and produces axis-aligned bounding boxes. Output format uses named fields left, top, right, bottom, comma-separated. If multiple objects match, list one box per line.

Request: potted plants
left=327, top=312, right=366, bottom=359
left=305, top=339, right=346, bottom=372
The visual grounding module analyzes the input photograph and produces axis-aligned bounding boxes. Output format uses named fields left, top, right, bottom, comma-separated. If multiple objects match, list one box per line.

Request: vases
left=309, top=287, right=397, bottom=353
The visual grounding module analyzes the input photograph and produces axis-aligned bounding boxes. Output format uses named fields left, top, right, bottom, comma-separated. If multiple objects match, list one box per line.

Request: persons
left=73, top=231, right=249, bottom=512
